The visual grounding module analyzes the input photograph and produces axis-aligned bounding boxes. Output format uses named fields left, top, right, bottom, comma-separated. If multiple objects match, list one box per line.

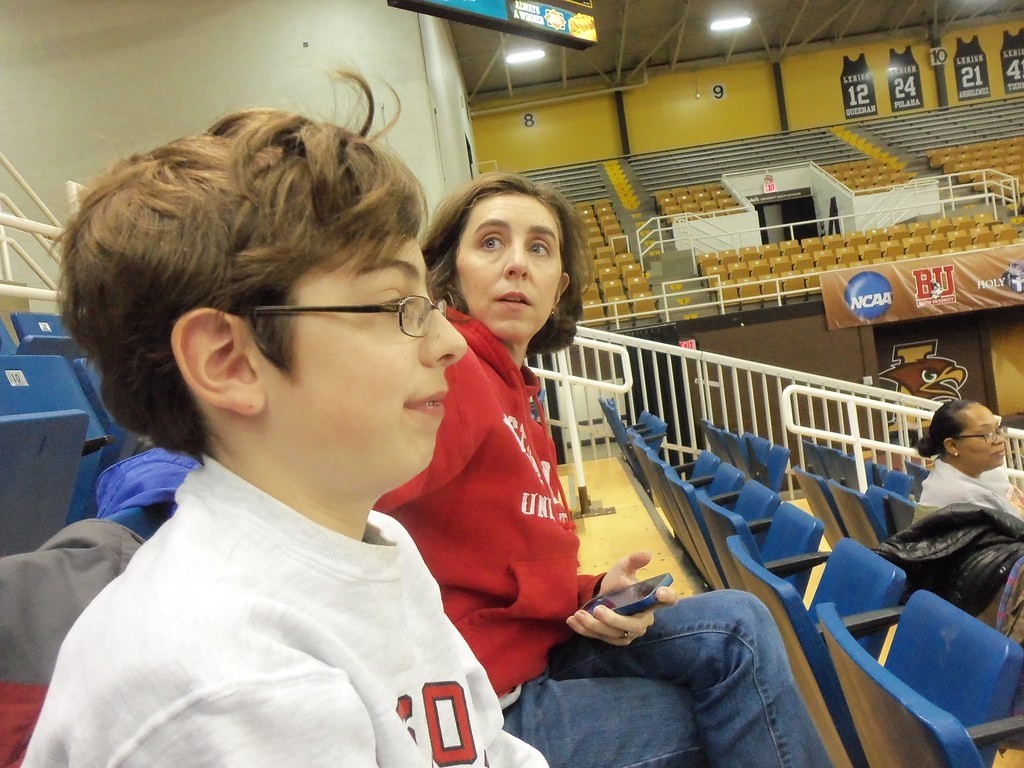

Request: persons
left=915, top=399, right=1024, bottom=524
left=14, top=113, right=551, bottom=768
left=369, top=173, right=831, bottom=768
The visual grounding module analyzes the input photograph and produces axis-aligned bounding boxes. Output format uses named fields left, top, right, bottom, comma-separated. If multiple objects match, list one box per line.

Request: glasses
left=949, top=425, right=1007, bottom=444
left=120, top=295, right=447, bottom=338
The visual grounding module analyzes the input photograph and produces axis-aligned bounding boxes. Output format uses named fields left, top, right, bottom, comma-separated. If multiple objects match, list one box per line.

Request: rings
left=621, top=632, right=632, bottom=640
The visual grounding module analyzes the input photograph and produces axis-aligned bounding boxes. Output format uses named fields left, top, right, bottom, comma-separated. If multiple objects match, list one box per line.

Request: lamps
left=710, top=16, right=751, bottom=30
left=505, top=46, right=545, bottom=63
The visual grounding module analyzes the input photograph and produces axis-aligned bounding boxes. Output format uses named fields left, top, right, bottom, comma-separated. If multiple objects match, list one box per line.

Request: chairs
left=0, top=311, right=136, bottom=553
left=569, top=135, right=1024, bottom=326
left=598, top=396, right=1024, bottom=768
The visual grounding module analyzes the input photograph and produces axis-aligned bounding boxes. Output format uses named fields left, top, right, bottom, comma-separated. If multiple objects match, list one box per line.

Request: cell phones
left=579, top=573, right=674, bottom=615
